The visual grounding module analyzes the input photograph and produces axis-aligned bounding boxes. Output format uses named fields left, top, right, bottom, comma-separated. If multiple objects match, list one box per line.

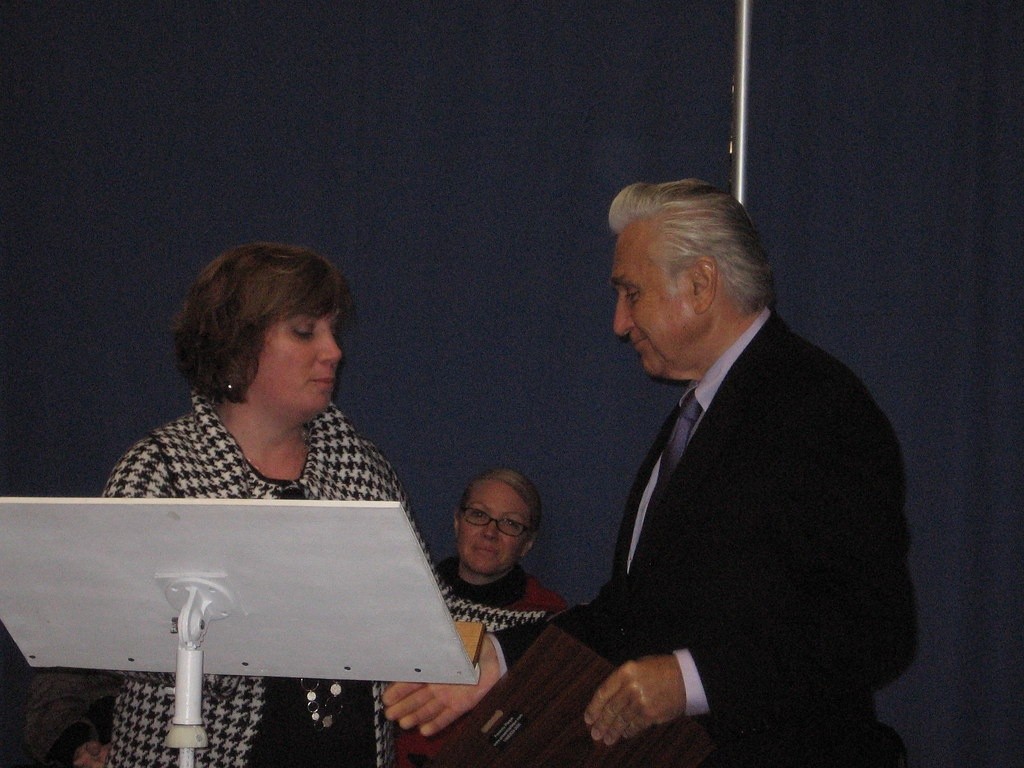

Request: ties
left=657, top=387, right=702, bottom=494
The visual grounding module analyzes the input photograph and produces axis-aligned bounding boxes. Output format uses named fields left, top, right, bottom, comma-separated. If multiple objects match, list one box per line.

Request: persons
left=20, top=668, right=119, bottom=768
left=382, top=176, right=919, bottom=768
left=100, top=241, right=589, bottom=768
left=436, top=468, right=567, bottom=614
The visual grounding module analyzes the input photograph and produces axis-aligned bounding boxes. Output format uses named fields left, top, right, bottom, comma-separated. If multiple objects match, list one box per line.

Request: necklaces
left=241, top=426, right=344, bottom=733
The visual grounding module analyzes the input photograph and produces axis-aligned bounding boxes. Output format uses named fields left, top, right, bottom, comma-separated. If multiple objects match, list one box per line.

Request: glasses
left=461, top=506, right=529, bottom=537
